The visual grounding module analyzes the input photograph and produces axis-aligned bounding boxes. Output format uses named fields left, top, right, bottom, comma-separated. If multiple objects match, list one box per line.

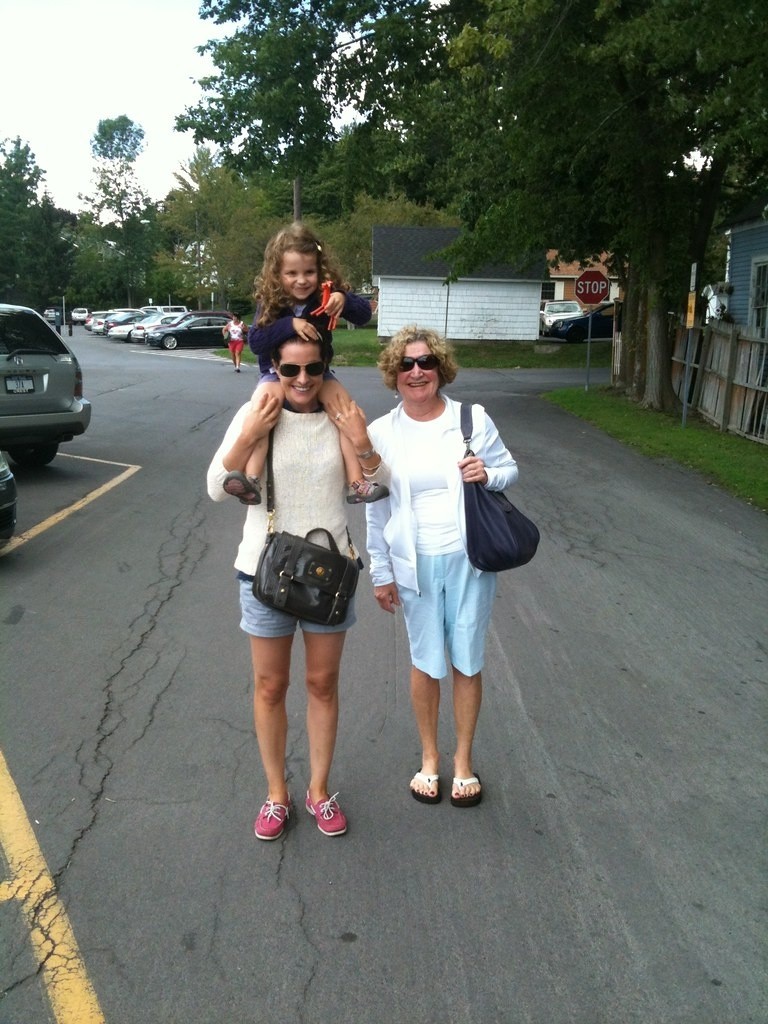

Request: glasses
left=277, top=362, right=324, bottom=377
left=399, top=354, right=439, bottom=372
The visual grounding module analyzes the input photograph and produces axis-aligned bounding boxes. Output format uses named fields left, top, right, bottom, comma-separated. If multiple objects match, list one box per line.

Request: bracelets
left=357, top=446, right=374, bottom=459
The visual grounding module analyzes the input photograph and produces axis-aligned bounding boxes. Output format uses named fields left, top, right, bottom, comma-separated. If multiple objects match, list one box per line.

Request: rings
left=474, top=470, right=476, bottom=475
left=335, top=412, right=341, bottom=419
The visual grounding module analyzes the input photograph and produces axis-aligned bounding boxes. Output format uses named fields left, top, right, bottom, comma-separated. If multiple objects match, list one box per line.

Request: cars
left=550, top=302, right=623, bottom=344
left=84, top=307, right=249, bottom=350
left=539, top=300, right=584, bottom=337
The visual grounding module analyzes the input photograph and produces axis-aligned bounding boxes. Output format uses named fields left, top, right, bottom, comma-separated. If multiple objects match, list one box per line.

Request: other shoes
left=235, top=369, right=241, bottom=373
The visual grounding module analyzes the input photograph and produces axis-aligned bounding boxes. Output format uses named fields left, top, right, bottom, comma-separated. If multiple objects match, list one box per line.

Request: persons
left=222, top=312, right=248, bottom=373
left=222, top=221, right=390, bottom=503
left=206, top=333, right=391, bottom=839
left=366, top=324, right=519, bottom=805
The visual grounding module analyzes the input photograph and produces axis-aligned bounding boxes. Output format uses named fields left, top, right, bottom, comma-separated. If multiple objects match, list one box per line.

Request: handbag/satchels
left=252, top=528, right=359, bottom=627
left=461, top=403, right=540, bottom=572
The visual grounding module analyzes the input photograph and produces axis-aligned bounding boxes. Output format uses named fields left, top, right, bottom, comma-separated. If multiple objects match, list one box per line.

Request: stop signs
left=574, top=270, right=610, bottom=306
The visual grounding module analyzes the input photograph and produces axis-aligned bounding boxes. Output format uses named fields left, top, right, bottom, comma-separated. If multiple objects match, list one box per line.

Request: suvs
left=0, top=303, right=92, bottom=468
left=43, top=307, right=89, bottom=325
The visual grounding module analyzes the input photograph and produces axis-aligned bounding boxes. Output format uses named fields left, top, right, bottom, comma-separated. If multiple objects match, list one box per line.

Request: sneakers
left=255, top=792, right=291, bottom=840
left=223, top=471, right=261, bottom=505
left=306, top=787, right=347, bottom=836
left=347, top=477, right=389, bottom=504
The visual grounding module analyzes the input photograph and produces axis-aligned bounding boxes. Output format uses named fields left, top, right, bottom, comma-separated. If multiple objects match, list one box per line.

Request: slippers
left=412, top=768, right=441, bottom=804
left=451, top=773, right=481, bottom=807
